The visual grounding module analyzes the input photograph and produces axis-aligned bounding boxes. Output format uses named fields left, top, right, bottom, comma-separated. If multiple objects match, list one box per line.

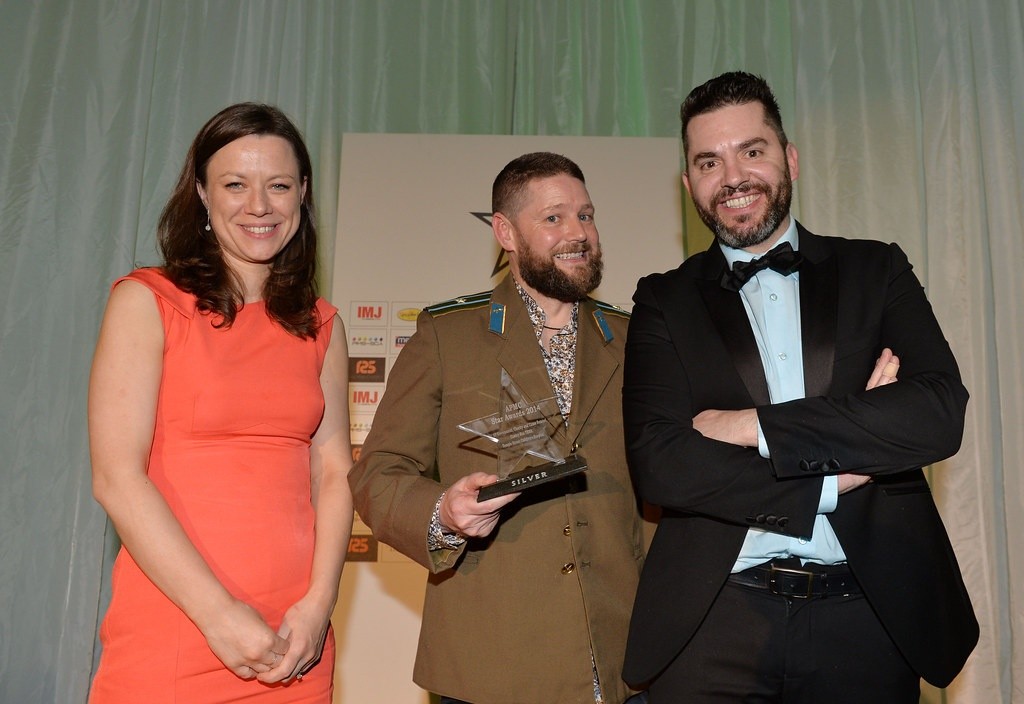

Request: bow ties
left=732, top=242, right=798, bottom=288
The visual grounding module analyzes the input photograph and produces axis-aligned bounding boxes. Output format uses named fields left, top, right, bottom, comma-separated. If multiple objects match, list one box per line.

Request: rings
left=297, top=673, right=302, bottom=679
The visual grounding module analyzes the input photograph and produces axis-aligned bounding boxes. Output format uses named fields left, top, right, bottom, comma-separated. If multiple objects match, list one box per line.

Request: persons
left=86, top=101, right=354, bottom=703
left=620, top=71, right=980, bottom=704
left=347, top=152, right=659, bottom=704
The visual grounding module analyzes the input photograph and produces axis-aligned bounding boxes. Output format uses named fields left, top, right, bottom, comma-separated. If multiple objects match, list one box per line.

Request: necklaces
left=544, top=325, right=561, bottom=330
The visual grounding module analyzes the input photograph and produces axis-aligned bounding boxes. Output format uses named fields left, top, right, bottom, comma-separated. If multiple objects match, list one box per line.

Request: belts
left=728, top=566, right=860, bottom=601
